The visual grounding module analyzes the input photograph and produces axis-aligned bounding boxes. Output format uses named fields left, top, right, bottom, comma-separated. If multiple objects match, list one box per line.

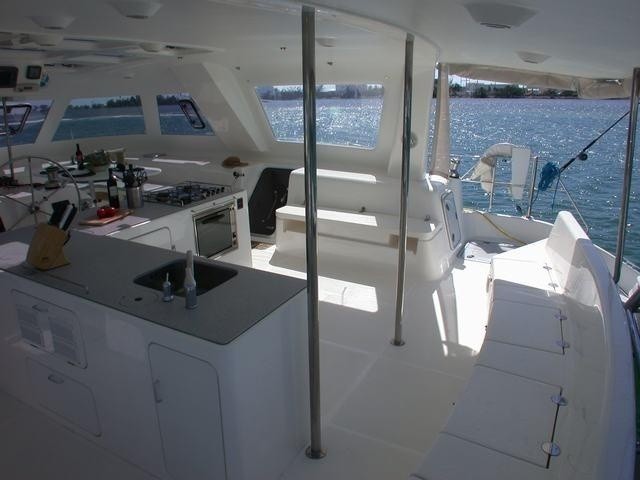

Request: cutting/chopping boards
left=78, top=207, right=133, bottom=227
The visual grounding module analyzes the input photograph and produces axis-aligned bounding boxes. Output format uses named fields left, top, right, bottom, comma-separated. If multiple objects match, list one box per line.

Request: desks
left=33, top=162, right=162, bottom=201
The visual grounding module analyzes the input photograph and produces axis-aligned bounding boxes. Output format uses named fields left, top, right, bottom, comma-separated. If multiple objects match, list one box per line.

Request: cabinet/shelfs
left=0, top=269, right=308, bottom=480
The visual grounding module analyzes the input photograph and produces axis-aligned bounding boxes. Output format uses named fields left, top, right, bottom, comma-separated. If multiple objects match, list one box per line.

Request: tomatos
left=107, top=206, right=116, bottom=216
left=97, top=208, right=106, bottom=218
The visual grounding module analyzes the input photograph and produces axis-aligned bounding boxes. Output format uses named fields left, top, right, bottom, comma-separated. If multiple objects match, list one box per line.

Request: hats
left=224, top=156, right=248, bottom=166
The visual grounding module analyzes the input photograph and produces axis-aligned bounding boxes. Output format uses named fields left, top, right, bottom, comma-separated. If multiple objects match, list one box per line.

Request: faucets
left=183, top=249, right=198, bottom=310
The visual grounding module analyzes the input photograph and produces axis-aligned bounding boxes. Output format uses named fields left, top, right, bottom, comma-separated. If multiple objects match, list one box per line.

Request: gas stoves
left=144, top=178, right=231, bottom=208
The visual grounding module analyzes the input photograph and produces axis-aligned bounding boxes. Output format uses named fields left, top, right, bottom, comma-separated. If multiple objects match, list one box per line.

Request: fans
left=0, top=156, right=81, bottom=233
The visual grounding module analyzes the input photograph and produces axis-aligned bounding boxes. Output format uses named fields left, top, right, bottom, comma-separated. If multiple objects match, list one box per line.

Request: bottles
left=125, top=164, right=138, bottom=187
left=107, top=168, right=120, bottom=209
left=75, top=144, right=84, bottom=169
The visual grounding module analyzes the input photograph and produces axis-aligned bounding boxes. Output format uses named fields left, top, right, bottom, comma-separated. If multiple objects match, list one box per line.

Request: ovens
left=192, top=190, right=253, bottom=268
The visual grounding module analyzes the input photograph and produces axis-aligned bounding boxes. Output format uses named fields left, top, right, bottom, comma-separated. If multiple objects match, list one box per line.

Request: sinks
left=131, top=258, right=240, bottom=298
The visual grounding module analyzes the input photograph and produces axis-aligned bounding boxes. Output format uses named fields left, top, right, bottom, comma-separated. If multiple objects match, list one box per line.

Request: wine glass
left=105, top=150, right=118, bottom=168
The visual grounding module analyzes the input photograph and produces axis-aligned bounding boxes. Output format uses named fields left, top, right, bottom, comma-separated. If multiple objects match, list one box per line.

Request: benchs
left=1, top=156, right=266, bottom=203
left=274, top=165, right=442, bottom=282
